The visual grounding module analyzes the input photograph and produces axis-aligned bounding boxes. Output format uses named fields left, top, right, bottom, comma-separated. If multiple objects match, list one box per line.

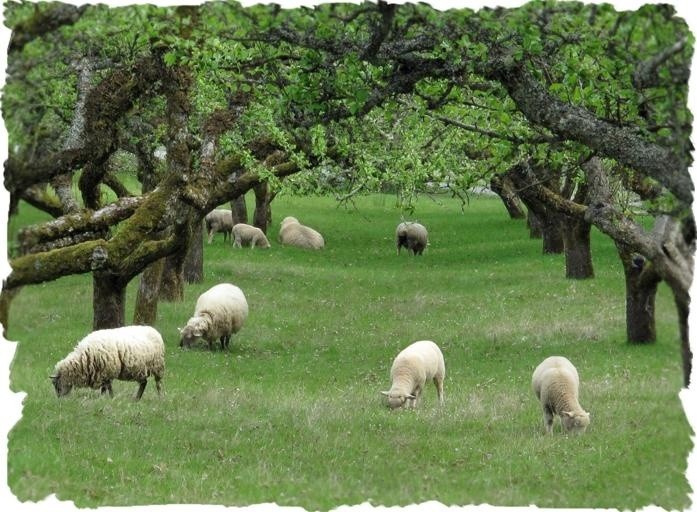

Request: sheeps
left=232, top=223, right=271, bottom=249
left=176, top=283, right=249, bottom=352
left=47, top=325, right=166, bottom=402
left=379, top=340, right=446, bottom=410
left=395, top=221, right=431, bottom=256
left=278, top=216, right=325, bottom=250
left=204, top=209, right=234, bottom=244
left=530, top=355, right=591, bottom=437
